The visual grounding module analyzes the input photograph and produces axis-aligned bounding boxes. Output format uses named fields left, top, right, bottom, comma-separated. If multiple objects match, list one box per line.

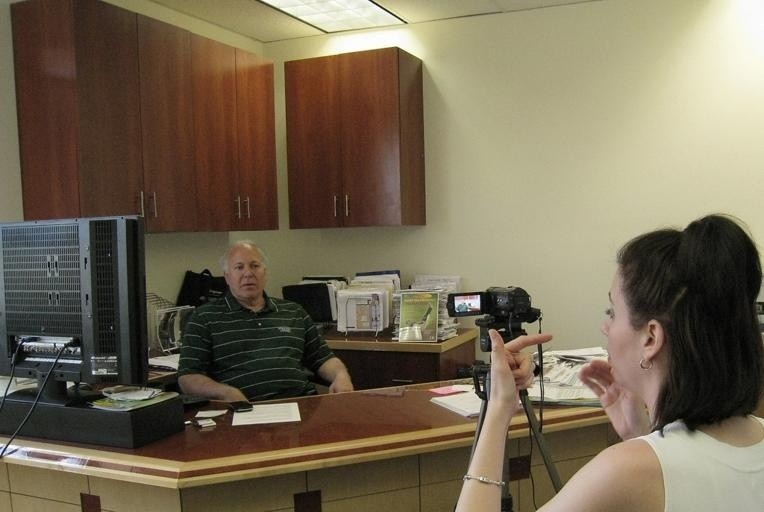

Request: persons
left=451, top=209, right=764, bottom=511
left=175, top=239, right=355, bottom=402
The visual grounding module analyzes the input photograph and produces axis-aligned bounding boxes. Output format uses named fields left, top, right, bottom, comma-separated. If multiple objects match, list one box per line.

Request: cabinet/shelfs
left=332, top=339, right=475, bottom=391
left=284, top=47, right=427, bottom=230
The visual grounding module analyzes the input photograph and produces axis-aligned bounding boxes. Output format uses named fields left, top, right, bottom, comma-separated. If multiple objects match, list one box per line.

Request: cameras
left=445, top=286, right=541, bottom=353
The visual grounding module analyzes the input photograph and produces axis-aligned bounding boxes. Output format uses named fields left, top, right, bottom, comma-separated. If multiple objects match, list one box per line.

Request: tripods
left=468, top=360, right=563, bottom=512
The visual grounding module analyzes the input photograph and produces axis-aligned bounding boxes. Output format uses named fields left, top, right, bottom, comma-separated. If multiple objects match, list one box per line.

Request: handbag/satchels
left=175, top=269, right=226, bottom=309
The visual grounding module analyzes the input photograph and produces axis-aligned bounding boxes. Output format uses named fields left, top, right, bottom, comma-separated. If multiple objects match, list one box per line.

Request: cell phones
left=228, top=401, right=253, bottom=412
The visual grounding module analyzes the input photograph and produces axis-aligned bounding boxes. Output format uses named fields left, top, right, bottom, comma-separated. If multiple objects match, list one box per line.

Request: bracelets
left=462, top=474, right=505, bottom=487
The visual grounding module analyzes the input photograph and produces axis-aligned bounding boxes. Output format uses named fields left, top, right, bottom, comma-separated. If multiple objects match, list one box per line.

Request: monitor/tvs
left=1, top=214, right=146, bottom=407
